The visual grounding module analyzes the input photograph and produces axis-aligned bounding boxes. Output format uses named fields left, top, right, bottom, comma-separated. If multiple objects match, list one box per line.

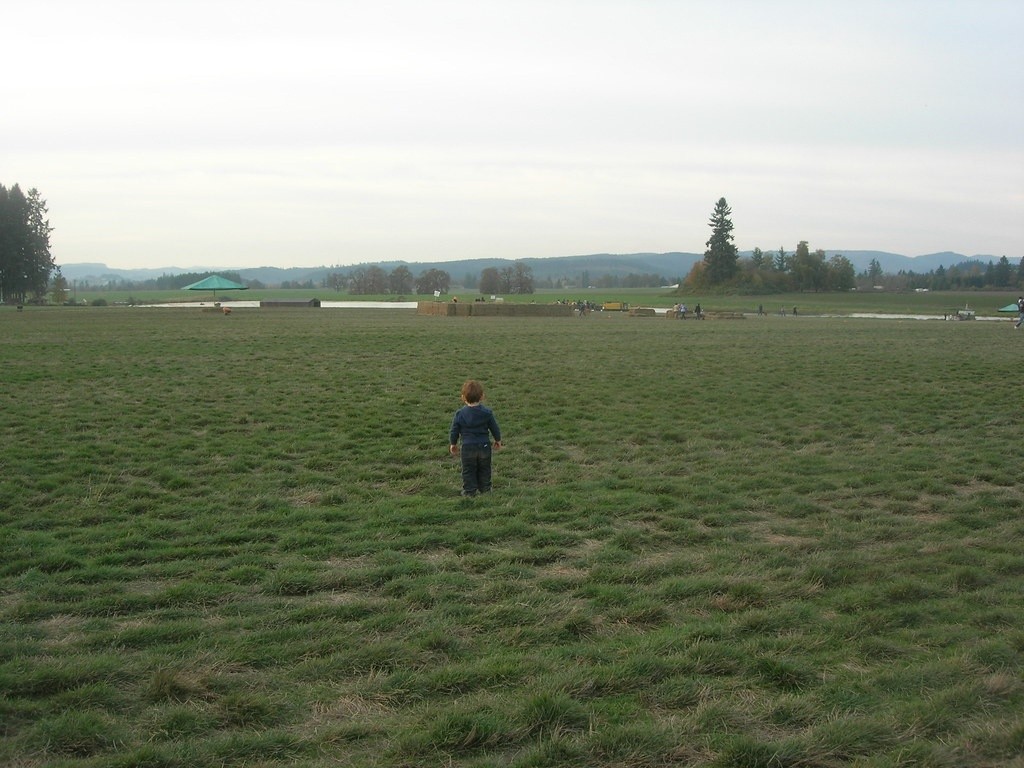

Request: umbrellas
left=180, top=275, right=249, bottom=298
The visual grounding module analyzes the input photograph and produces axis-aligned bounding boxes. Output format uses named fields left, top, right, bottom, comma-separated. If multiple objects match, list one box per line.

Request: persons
left=1014, top=296, right=1024, bottom=329
left=792, top=305, right=800, bottom=316
left=554, top=298, right=606, bottom=317
left=529, top=300, right=535, bottom=304
left=674, top=302, right=686, bottom=321
left=449, top=380, right=502, bottom=497
left=695, top=303, right=703, bottom=320
left=757, top=305, right=765, bottom=315
